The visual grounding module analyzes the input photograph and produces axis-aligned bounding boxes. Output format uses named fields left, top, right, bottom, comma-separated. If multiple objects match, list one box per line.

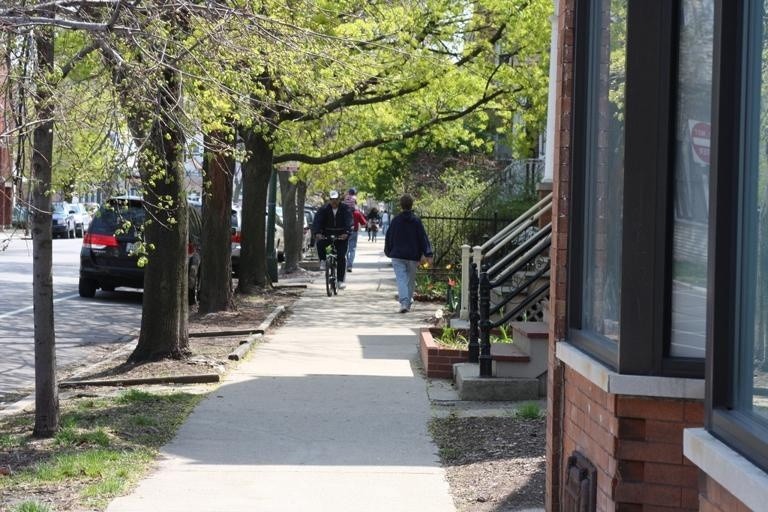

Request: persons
left=311, top=190, right=355, bottom=290
left=344, top=188, right=364, bottom=215
left=384, top=194, right=433, bottom=313
left=365, top=208, right=390, bottom=242
left=346, top=210, right=366, bottom=272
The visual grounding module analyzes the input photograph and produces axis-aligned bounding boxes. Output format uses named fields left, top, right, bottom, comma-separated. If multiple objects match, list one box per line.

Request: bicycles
left=323, top=235, right=344, bottom=297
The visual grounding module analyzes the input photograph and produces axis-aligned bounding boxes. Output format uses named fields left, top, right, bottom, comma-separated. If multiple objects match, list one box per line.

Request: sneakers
left=338, top=281, right=346, bottom=289
left=320, top=259, right=326, bottom=270
left=400, top=302, right=410, bottom=313
left=347, top=267, right=353, bottom=272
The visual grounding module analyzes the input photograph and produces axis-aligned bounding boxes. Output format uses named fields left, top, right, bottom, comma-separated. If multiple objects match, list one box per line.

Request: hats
left=349, top=189, right=355, bottom=193
left=330, top=191, right=340, bottom=199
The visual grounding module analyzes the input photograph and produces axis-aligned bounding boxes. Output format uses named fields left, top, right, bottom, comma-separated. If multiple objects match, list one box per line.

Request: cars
left=79, top=194, right=203, bottom=304
left=232, top=203, right=318, bottom=277
left=50, top=202, right=101, bottom=239
